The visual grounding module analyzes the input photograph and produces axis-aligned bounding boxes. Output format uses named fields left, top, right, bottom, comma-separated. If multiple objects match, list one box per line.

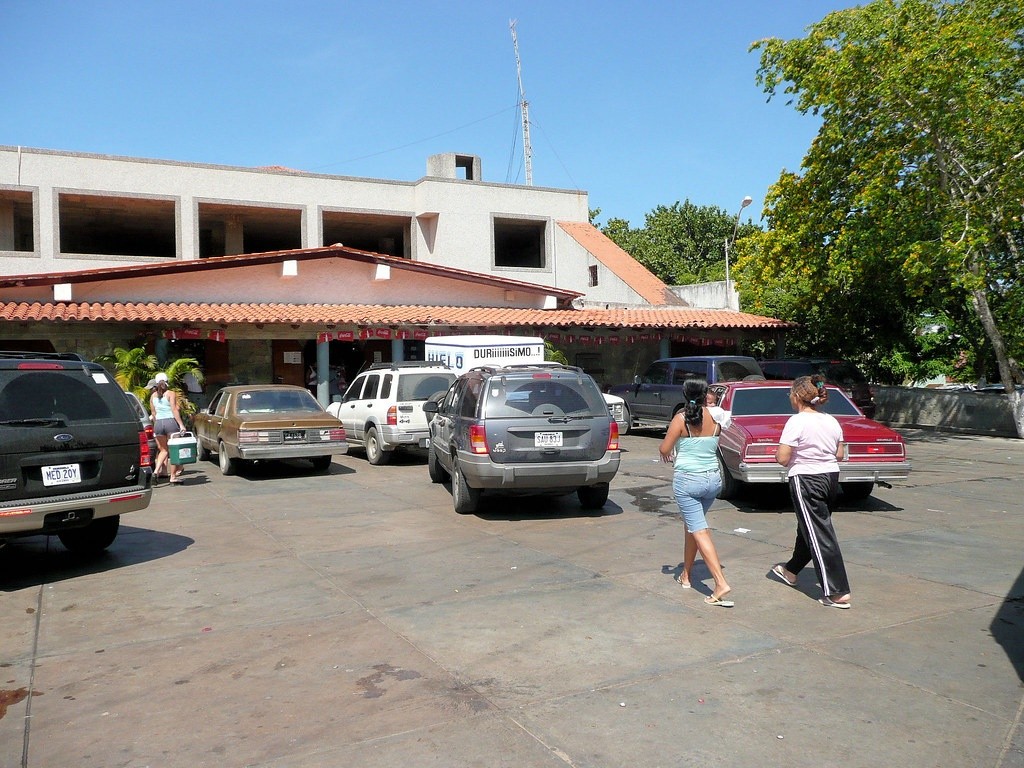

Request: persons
left=145, top=374, right=186, bottom=485
left=329, top=366, right=346, bottom=401
left=306, top=362, right=317, bottom=396
left=771, top=375, right=851, bottom=608
left=182, top=362, right=206, bottom=414
left=659, top=376, right=735, bottom=606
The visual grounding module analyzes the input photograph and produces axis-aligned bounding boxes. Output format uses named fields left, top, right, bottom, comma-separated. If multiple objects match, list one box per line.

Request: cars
left=124, top=392, right=156, bottom=462
left=706, top=379, right=911, bottom=497
left=193, top=385, right=350, bottom=475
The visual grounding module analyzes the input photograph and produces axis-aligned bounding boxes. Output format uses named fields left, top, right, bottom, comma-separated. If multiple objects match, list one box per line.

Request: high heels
left=151, top=473, right=158, bottom=485
left=170, top=478, right=185, bottom=486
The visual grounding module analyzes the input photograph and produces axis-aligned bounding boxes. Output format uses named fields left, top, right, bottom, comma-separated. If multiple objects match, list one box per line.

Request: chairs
left=529, top=391, right=555, bottom=409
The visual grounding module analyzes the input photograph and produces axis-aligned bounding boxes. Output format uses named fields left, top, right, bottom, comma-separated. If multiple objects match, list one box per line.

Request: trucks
left=424, top=335, right=628, bottom=436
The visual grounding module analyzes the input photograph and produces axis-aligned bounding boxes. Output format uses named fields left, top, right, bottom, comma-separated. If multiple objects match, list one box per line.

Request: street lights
left=725, top=197, right=752, bottom=308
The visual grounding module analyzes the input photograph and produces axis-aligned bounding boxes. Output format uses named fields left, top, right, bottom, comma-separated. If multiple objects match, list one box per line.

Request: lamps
left=145, top=324, right=153, bottom=329
left=255, top=323, right=264, bottom=329
left=420, top=326, right=430, bottom=331
left=291, top=325, right=300, bottom=329
left=220, top=324, right=228, bottom=329
left=503, top=325, right=518, bottom=331
left=358, top=325, right=368, bottom=329
left=559, top=326, right=786, bottom=334
left=533, top=325, right=545, bottom=331
left=183, top=324, right=192, bottom=328
left=478, top=326, right=487, bottom=331
left=327, top=325, right=336, bottom=329
left=450, top=326, right=459, bottom=330
left=388, top=325, right=400, bottom=330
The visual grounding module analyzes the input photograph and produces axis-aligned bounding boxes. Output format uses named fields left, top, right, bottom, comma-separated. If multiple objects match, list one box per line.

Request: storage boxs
left=167, top=437, right=198, bottom=466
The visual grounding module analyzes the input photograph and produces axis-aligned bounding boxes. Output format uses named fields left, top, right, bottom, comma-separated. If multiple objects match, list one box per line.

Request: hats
left=156, top=373, right=168, bottom=383
left=143, top=379, right=157, bottom=389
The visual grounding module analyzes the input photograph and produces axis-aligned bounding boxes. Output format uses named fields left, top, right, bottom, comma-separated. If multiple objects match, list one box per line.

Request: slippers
left=677, top=576, right=691, bottom=588
left=818, top=595, right=851, bottom=608
left=175, top=468, right=184, bottom=476
left=771, top=565, right=797, bottom=585
left=704, top=594, right=734, bottom=606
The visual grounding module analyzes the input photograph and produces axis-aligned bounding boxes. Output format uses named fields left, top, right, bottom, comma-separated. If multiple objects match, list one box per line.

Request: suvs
left=325, top=360, right=460, bottom=465
left=0, top=351, right=153, bottom=554
left=758, top=359, right=875, bottom=420
left=427, top=364, right=621, bottom=514
left=607, top=356, right=767, bottom=430
